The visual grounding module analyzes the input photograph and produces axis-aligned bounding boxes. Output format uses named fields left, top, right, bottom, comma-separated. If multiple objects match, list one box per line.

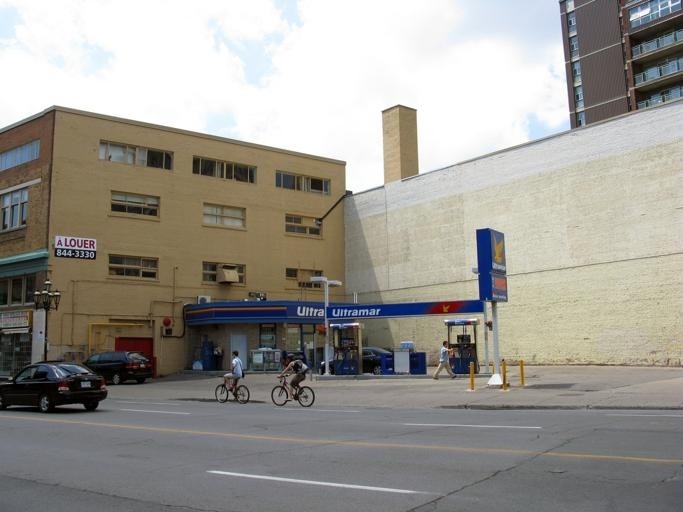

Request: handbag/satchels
left=242, top=372, right=244, bottom=378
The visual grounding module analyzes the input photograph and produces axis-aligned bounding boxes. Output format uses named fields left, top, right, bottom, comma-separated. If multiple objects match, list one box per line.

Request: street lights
left=33, top=279, right=61, bottom=361
left=310, top=277, right=343, bottom=376
left=472, top=268, right=490, bottom=373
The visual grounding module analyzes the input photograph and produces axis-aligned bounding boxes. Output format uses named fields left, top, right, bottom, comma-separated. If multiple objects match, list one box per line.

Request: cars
left=319, top=347, right=393, bottom=375
left=82, top=351, right=154, bottom=385
left=0, top=360, right=108, bottom=413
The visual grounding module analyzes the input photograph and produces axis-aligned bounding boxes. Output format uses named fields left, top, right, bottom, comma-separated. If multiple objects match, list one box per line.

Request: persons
left=223, top=350, right=243, bottom=402
left=277, top=352, right=309, bottom=403
left=431, top=340, right=457, bottom=380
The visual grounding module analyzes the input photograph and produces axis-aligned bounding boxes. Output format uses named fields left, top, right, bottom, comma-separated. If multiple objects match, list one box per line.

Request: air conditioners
left=196, top=294, right=211, bottom=305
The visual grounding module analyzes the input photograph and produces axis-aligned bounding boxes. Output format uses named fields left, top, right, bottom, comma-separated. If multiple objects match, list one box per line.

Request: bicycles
left=214, top=375, right=249, bottom=404
left=270, top=374, right=315, bottom=408
